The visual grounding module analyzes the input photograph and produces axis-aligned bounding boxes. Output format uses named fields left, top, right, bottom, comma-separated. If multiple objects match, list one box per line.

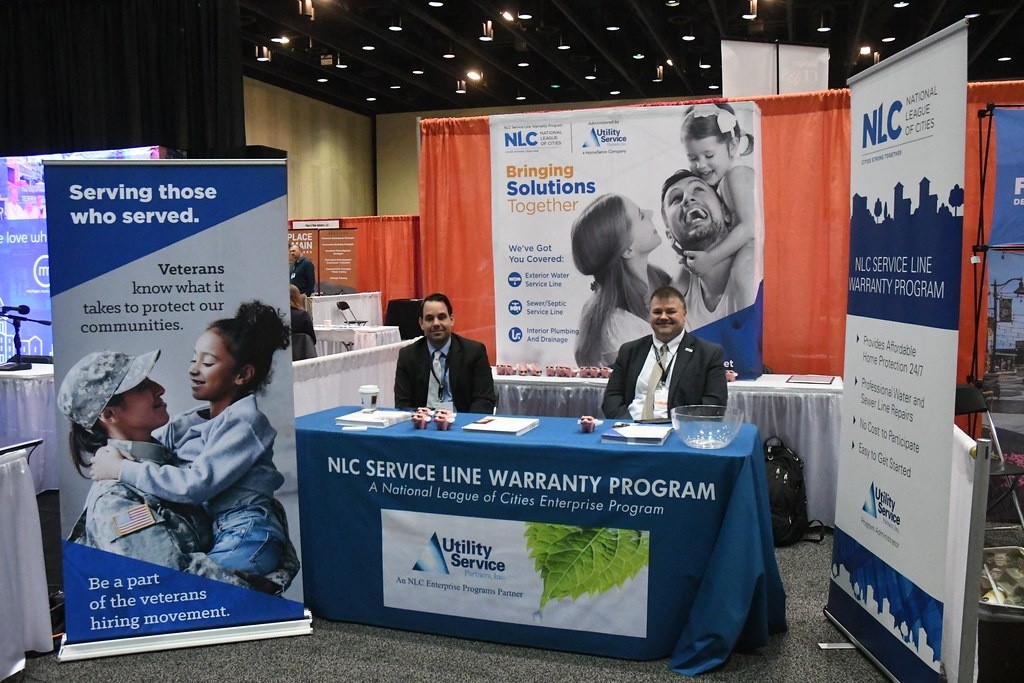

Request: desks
left=307, top=292, right=383, bottom=326
left=0, top=365, right=61, bottom=496
left=311, top=324, right=401, bottom=352
left=489, top=375, right=844, bottom=529
left=294, top=405, right=787, bottom=674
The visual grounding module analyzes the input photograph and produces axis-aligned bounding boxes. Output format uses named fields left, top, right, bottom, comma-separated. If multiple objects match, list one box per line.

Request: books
left=462, top=416, right=540, bottom=436
left=335, top=408, right=412, bottom=429
left=600, top=426, right=674, bottom=447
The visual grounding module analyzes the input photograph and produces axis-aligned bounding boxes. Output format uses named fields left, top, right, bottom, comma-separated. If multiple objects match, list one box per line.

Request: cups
left=359, top=385, right=380, bottom=410
left=324, top=320, right=332, bottom=329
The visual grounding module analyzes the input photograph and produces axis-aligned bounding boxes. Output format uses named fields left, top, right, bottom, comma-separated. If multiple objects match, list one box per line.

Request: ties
left=641, top=344, right=668, bottom=421
left=427, top=350, right=443, bottom=408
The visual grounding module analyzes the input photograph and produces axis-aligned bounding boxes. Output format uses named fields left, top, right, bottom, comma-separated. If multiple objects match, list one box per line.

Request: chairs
left=337, top=300, right=369, bottom=327
left=955, top=386, right=1024, bottom=535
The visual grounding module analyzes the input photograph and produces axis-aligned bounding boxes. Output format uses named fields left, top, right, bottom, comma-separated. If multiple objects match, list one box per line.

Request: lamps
left=441, top=47, right=456, bottom=59
left=389, top=13, right=402, bottom=32
left=666, top=0, right=759, bottom=90
left=412, top=64, right=424, bottom=75
left=476, top=19, right=495, bottom=42
left=609, top=82, right=622, bottom=96
left=455, top=78, right=469, bottom=95
left=256, top=45, right=271, bottom=61
left=429, top=0, right=444, bottom=7
left=390, top=80, right=401, bottom=90
left=317, top=73, right=328, bottom=83
left=651, top=64, right=665, bottom=84
left=549, top=78, right=563, bottom=89
left=334, top=53, right=346, bottom=69
left=514, top=84, right=526, bottom=102
left=365, top=91, right=377, bottom=101
left=361, top=36, right=376, bottom=51
left=296, top=0, right=316, bottom=23
left=517, top=3, right=649, bottom=78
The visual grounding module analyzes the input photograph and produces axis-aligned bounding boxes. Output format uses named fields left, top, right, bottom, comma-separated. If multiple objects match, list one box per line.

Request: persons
left=289, top=244, right=315, bottom=297
left=56, top=299, right=300, bottom=598
left=289, top=284, right=317, bottom=344
left=602, top=286, right=729, bottom=420
left=570, top=100, right=756, bottom=363
left=395, top=292, right=495, bottom=414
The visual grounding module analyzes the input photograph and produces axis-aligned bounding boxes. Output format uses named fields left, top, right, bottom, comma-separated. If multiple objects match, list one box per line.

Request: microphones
left=2, top=305, right=30, bottom=315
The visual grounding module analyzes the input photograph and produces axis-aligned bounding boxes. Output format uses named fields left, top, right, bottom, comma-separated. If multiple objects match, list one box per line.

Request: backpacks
left=760, top=435, right=810, bottom=546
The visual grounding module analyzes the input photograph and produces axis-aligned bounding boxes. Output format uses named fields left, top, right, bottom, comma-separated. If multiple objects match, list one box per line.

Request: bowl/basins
left=670, top=404, right=744, bottom=449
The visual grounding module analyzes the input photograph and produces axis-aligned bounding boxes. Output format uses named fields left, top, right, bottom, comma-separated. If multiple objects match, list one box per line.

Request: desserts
left=577, top=416, right=596, bottom=432
left=496, top=362, right=542, bottom=376
left=546, top=366, right=578, bottom=377
left=411, top=407, right=457, bottom=431
left=579, top=366, right=613, bottom=378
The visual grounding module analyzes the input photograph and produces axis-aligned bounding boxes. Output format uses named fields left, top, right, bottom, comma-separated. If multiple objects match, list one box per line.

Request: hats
left=58, top=349, right=162, bottom=430
